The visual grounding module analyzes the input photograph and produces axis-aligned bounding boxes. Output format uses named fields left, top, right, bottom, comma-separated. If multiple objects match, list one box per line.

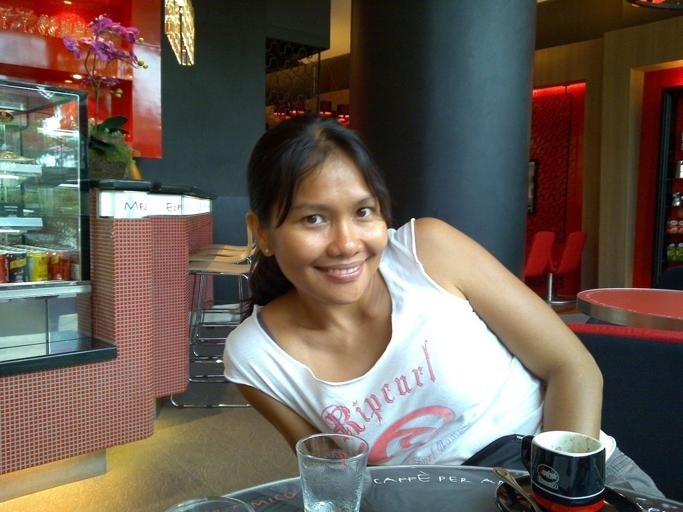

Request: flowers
left=63, top=15, right=148, bottom=125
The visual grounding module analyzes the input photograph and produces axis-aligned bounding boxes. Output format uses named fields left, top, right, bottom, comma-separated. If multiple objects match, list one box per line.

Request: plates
left=496, top=474, right=643, bottom=512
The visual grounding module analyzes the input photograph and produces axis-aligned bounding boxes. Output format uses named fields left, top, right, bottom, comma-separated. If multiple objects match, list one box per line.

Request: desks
left=186, top=464, right=683, bottom=511
left=577, top=288, right=683, bottom=329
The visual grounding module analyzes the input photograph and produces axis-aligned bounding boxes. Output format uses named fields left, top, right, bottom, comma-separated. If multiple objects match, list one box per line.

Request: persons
left=218, top=110, right=664, bottom=499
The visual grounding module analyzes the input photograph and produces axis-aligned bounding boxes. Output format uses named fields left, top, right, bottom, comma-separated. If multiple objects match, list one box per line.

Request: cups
left=296, top=431, right=370, bottom=512
left=520, top=431, right=606, bottom=511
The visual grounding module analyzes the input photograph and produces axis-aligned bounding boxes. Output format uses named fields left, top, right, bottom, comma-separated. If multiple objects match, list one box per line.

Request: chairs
left=526, top=232, right=556, bottom=281
left=544, top=233, right=587, bottom=306
left=565, top=322, right=683, bottom=502
left=170, top=226, right=256, bottom=409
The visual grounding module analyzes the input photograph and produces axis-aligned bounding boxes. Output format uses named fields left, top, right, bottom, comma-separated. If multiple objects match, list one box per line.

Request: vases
left=88, top=125, right=133, bottom=181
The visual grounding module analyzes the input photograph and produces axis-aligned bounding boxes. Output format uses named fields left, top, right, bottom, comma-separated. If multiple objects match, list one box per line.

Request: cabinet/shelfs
left=0, top=80, right=90, bottom=291
left=651, top=87, right=682, bottom=290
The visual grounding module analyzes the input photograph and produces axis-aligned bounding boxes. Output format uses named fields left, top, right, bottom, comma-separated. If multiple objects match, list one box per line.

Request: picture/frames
left=527, top=160, right=537, bottom=216
